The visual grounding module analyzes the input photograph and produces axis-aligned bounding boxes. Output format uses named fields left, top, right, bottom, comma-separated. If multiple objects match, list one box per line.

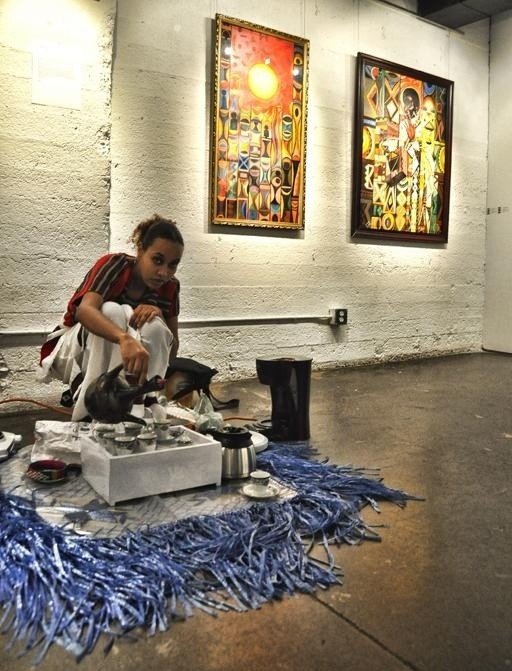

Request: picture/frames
left=206, top=12, right=311, bottom=233
left=351, top=52, right=455, bottom=245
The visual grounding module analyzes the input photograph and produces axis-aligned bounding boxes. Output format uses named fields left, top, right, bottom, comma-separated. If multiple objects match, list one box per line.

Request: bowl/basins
left=27, top=459, right=67, bottom=483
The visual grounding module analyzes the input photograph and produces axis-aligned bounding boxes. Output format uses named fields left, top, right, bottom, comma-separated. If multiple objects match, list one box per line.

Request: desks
left=77, top=424, right=221, bottom=507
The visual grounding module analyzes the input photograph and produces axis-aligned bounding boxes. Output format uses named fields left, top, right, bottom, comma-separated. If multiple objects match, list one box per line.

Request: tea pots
left=84, top=360, right=168, bottom=427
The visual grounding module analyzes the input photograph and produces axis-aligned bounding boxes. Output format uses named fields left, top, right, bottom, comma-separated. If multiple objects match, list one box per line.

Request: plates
left=242, top=484, right=279, bottom=500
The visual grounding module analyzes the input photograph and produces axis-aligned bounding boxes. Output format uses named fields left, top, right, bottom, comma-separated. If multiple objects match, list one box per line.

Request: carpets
left=0, top=437, right=428, bottom=667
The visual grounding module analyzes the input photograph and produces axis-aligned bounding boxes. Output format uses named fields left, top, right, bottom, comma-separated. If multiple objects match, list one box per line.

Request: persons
left=33, top=213, right=185, bottom=424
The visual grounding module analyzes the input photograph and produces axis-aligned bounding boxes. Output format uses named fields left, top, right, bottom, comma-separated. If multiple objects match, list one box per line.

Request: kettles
left=202, top=426, right=256, bottom=480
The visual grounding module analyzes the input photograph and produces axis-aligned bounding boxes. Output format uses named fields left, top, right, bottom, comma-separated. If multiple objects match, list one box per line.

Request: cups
left=93, top=427, right=115, bottom=441
left=145, top=418, right=171, bottom=438
left=102, top=432, right=124, bottom=453
left=250, top=470, right=270, bottom=493
left=136, top=433, right=157, bottom=451
left=114, top=436, right=137, bottom=456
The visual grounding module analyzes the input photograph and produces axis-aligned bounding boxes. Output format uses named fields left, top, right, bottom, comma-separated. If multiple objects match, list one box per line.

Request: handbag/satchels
left=163, top=358, right=219, bottom=402
left=30, top=420, right=97, bottom=465
